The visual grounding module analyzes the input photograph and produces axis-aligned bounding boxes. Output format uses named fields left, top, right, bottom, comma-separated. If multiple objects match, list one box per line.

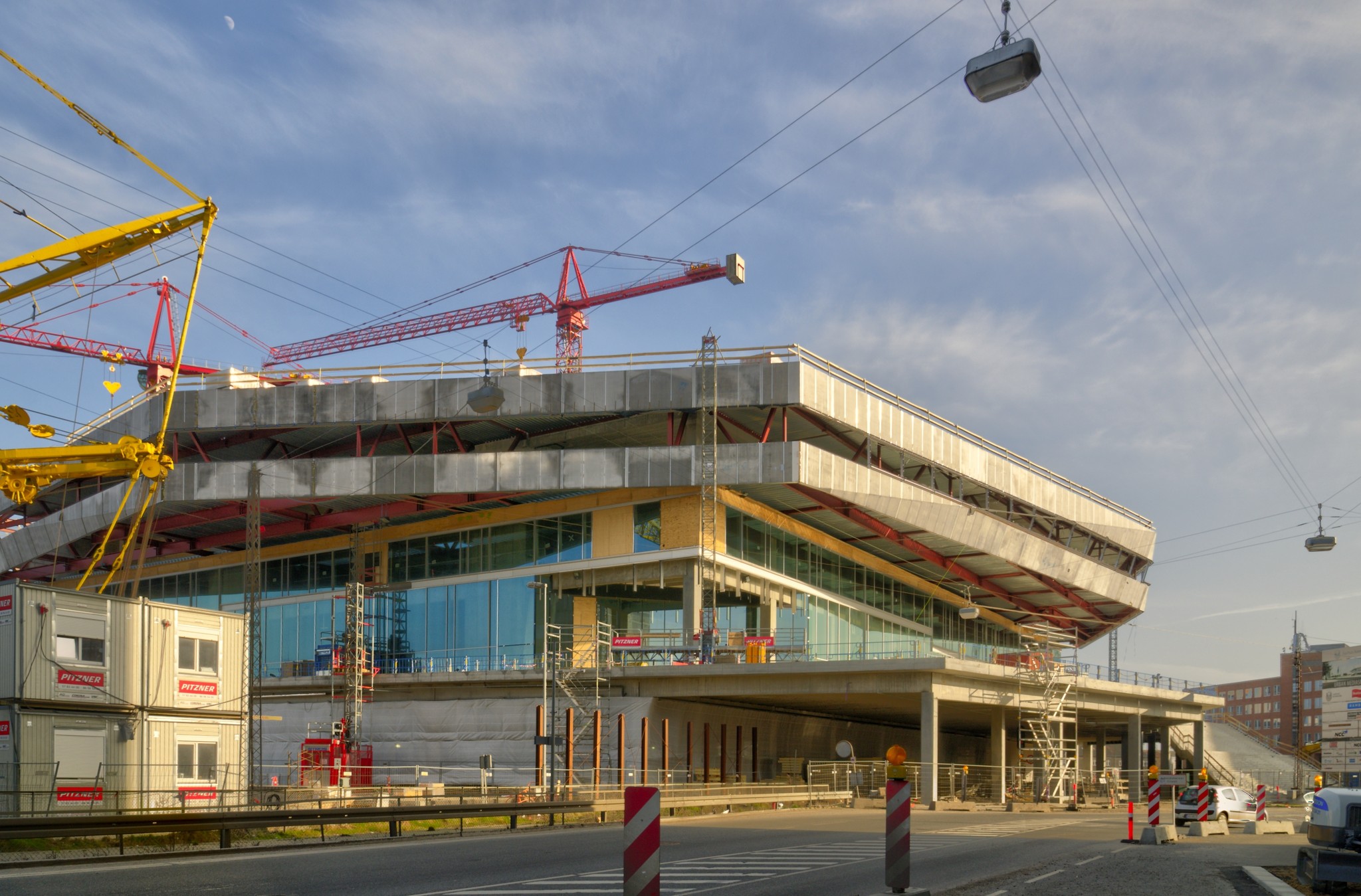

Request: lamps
left=743, top=575, right=750, bottom=583
left=959, top=586, right=980, bottom=618
left=573, top=572, right=580, bottom=580
left=1305, top=503, right=1337, bottom=551
left=963, top=0, right=1041, bottom=102
left=119, top=718, right=140, bottom=729
left=466, top=339, right=505, bottom=413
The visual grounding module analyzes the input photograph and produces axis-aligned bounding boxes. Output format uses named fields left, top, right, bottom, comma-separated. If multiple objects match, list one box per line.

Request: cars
left=1174, top=784, right=1269, bottom=827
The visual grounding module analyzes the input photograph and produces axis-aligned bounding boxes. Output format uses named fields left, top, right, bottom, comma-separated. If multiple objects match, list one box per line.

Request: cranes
left=1, top=276, right=331, bottom=388
left=690, top=326, right=730, bottom=642
left=260, top=243, right=746, bottom=375
